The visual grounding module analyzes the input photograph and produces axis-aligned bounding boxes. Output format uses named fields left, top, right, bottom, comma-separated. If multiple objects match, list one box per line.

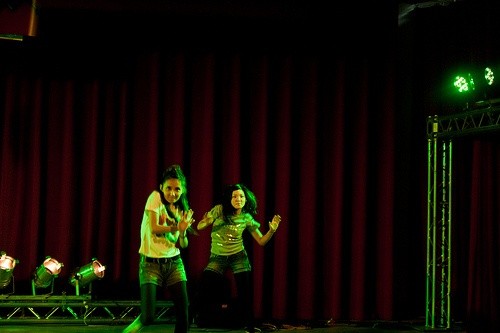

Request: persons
left=186, top=183, right=282, bottom=333
left=138, top=164, right=196, bottom=333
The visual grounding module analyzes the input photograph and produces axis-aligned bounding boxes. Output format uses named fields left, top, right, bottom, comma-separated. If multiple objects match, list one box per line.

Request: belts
left=146, top=255, right=180, bottom=264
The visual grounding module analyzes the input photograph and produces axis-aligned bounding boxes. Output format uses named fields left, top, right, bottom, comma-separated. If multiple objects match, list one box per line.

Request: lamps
left=32, top=256, right=62, bottom=289
left=0, top=255, right=16, bottom=286
left=70, top=258, right=105, bottom=287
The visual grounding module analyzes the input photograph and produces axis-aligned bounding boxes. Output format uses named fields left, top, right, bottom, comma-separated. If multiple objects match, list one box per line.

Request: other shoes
left=244, top=324, right=261, bottom=333
left=190, top=319, right=208, bottom=328
